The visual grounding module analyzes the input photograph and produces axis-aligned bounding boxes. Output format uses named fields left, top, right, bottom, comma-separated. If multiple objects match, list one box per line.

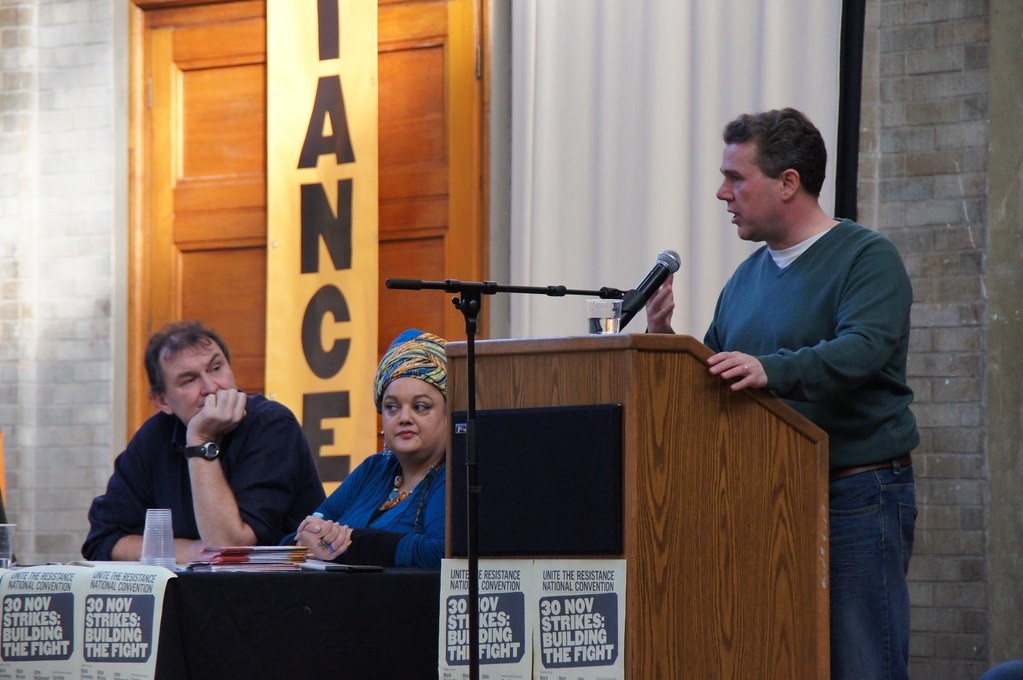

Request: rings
left=744, top=365, right=748, bottom=374
left=317, top=538, right=328, bottom=551
left=328, top=544, right=334, bottom=552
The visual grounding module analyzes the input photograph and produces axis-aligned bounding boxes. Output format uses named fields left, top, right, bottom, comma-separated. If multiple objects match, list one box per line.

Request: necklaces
left=384, top=475, right=408, bottom=511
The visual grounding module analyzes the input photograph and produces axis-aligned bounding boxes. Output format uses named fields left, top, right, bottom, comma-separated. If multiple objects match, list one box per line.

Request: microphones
left=614, top=249, right=681, bottom=332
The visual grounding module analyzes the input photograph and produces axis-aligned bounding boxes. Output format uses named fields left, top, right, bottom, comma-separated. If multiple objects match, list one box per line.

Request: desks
left=0, top=573, right=438, bottom=680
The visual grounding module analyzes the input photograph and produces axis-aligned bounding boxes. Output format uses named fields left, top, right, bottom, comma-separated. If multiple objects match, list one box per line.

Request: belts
left=829, top=452, right=912, bottom=484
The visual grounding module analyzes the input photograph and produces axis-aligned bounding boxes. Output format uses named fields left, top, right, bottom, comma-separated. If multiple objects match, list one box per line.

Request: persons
left=645, top=110, right=921, bottom=680
left=278, top=329, right=447, bottom=569
left=80, top=320, right=326, bottom=564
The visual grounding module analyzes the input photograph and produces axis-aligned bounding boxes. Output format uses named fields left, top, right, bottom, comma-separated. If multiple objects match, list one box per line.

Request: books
left=193, top=546, right=309, bottom=572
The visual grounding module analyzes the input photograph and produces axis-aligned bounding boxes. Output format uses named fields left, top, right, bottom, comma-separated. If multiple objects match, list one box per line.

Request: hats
left=374, top=328, right=448, bottom=412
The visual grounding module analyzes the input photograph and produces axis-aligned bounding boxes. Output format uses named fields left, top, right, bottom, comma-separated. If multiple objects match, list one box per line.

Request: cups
left=139, top=509, right=177, bottom=571
left=586, top=299, right=624, bottom=334
left=0, top=523, right=18, bottom=569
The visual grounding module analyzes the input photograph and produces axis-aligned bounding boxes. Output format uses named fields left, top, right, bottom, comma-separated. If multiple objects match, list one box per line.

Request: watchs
left=183, top=441, right=219, bottom=461
